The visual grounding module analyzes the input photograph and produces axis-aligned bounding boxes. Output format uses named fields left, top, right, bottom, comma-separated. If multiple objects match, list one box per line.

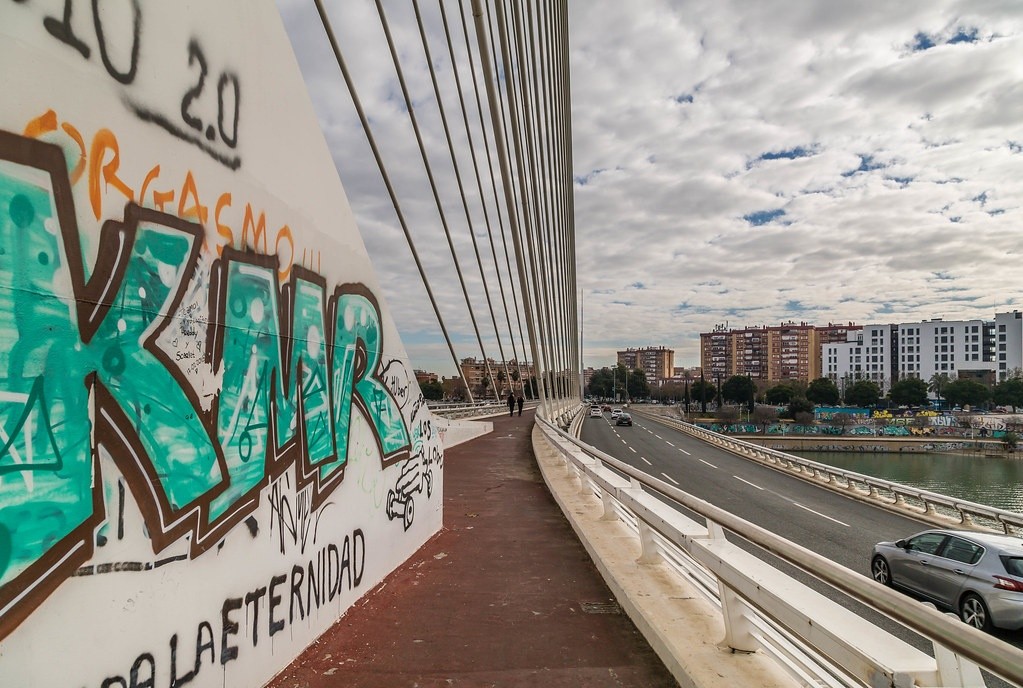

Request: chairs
left=948, top=541, right=973, bottom=558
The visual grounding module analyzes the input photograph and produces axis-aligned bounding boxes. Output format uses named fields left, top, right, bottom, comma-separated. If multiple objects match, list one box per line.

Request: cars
left=615, top=413, right=633, bottom=426
left=869, top=528, right=1023, bottom=635
left=611, top=409, right=623, bottom=419
left=588, top=402, right=612, bottom=419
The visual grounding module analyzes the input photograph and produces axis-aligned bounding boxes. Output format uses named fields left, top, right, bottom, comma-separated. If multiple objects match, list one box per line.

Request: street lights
left=625, top=365, right=630, bottom=404
left=613, top=368, right=618, bottom=406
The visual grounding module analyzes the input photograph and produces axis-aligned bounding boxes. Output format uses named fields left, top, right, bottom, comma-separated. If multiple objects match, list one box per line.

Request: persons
left=517, top=394, right=524, bottom=416
left=508, top=393, right=516, bottom=417
